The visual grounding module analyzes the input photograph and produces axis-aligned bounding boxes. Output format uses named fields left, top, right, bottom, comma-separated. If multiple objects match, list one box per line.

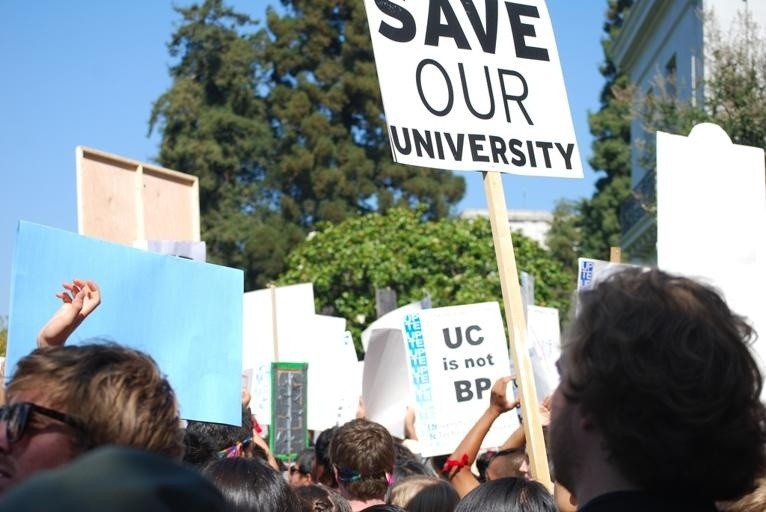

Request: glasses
left=289, top=465, right=307, bottom=476
left=0, top=401, right=87, bottom=444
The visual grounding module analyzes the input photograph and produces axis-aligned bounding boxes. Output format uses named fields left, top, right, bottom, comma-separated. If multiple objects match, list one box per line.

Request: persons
left=2, top=262, right=766, bottom=512
